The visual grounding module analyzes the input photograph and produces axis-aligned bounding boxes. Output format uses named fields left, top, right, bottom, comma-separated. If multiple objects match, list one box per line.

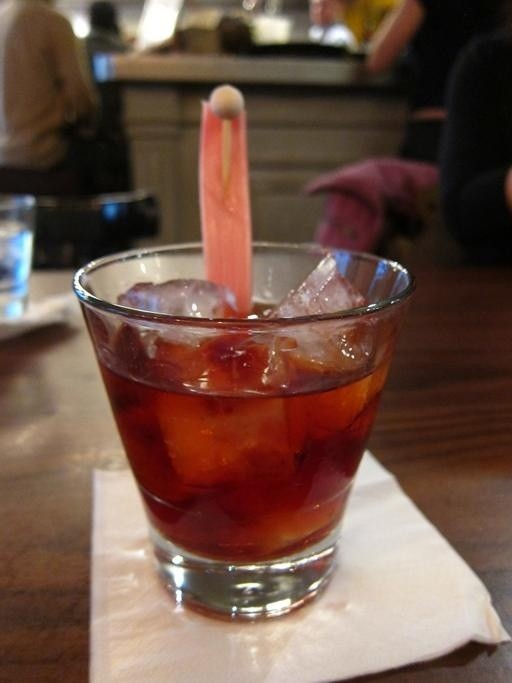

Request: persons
left=302, top=0, right=512, bottom=265
left=0, top=0, right=141, bottom=171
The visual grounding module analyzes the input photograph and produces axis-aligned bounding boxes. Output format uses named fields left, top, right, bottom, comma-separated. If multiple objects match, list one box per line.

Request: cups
left=0, top=193, right=34, bottom=322
left=71, top=241, right=416, bottom=624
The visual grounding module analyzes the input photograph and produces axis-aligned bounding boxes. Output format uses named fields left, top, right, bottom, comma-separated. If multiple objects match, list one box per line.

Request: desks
left=3, top=266, right=511, bottom=683
